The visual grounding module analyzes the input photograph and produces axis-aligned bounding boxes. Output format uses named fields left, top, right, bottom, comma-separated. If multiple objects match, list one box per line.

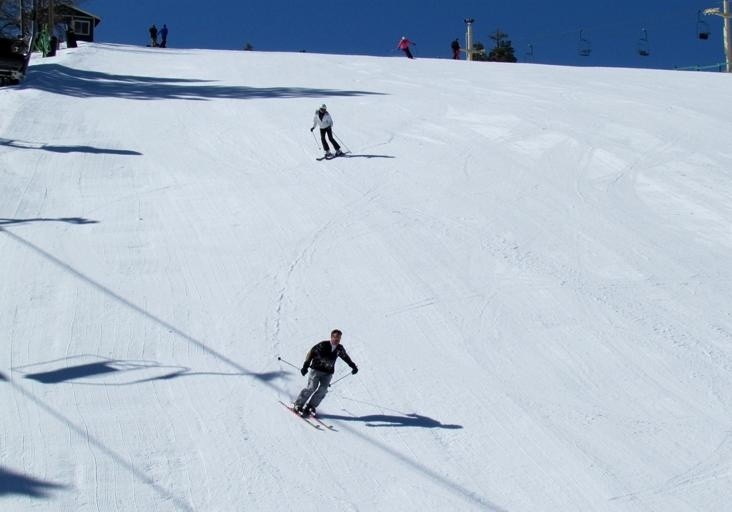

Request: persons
left=158, top=24, right=168, bottom=48
left=398, top=36, right=416, bottom=59
left=149, top=24, right=158, bottom=46
left=451, top=39, right=460, bottom=59
left=293, top=330, right=358, bottom=417
left=311, top=103, right=343, bottom=157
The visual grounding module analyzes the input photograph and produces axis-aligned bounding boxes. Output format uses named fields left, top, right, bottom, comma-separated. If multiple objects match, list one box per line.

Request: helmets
left=319, top=104, right=328, bottom=110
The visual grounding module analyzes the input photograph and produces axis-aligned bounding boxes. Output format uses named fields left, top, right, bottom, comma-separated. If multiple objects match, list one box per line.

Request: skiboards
left=280, top=400, right=333, bottom=429
left=316, top=152, right=348, bottom=161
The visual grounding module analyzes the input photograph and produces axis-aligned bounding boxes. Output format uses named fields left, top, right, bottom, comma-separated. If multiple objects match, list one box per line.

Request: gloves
left=349, top=362, right=358, bottom=374
left=300, top=362, right=310, bottom=376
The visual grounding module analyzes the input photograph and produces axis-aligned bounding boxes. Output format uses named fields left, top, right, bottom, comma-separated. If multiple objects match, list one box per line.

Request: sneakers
left=293, top=404, right=318, bottom=417
